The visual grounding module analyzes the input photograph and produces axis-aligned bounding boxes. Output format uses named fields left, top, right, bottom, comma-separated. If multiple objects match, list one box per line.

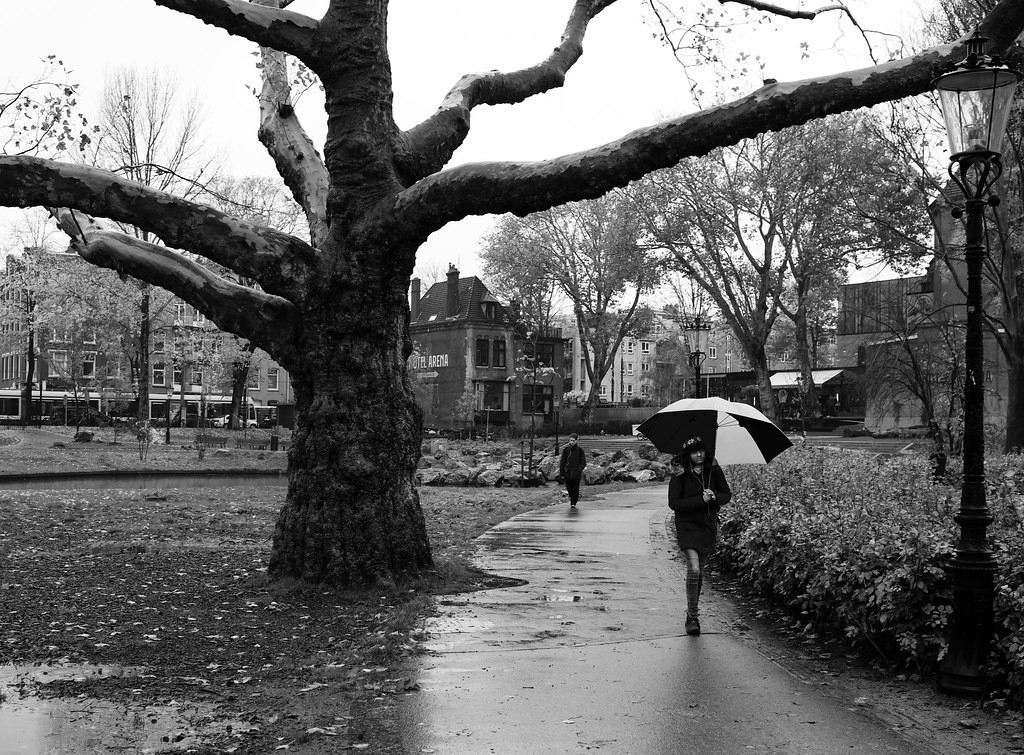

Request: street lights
left=164, top=385, right=175, bottom=444
left=678, top=312, right=713, bottom=399
left=928, top=23, right=1024, bottom=697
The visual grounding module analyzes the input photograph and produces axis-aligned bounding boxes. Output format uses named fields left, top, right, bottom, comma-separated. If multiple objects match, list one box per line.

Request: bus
left=0, top=386, right=257, bottom=421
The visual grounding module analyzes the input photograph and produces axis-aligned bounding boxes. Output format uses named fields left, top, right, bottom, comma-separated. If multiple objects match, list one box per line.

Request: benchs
left=193, top=435, right=226, bottom=448
left=234, top=438, right=270, bottom=451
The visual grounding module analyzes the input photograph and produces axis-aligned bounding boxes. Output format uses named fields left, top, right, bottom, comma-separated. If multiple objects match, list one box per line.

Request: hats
left=682, top=433, right=706, bottom=451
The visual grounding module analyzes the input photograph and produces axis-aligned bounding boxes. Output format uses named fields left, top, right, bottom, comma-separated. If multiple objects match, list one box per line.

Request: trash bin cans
left=270, top=435, right=278, bottom=451
left=460, top=428, right=469, bottom=440
left=447, top=430, right=461, bottom=441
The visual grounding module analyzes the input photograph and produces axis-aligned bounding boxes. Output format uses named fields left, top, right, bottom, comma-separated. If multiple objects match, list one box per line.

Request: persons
left=560, top=432, right=586, bottom=508
left=668, top=435, right=732, bottom=635
left=777, top=388, right=839, bottom=420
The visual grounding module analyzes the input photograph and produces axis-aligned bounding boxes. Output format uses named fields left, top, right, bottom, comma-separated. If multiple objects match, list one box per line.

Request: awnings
left=756, top=370, right=843, bottom=390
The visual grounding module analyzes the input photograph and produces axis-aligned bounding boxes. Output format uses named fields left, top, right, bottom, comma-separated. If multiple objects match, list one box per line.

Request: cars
left=214, top=414, right=260, bottom=429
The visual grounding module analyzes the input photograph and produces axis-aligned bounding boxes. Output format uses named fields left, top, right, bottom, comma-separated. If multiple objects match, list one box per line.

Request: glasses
left=690, top=449, right=706, bottom=454
left=570, top=440, right=576, bottom=441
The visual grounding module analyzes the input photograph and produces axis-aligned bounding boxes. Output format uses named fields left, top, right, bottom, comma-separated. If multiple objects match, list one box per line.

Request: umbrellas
left=634, top=395, right=796, bottom=517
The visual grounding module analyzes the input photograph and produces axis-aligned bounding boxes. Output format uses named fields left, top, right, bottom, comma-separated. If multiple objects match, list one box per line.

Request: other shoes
left=685, top=615, right=700, bottom=636
left=571, top=505, right=575, bottom=508
